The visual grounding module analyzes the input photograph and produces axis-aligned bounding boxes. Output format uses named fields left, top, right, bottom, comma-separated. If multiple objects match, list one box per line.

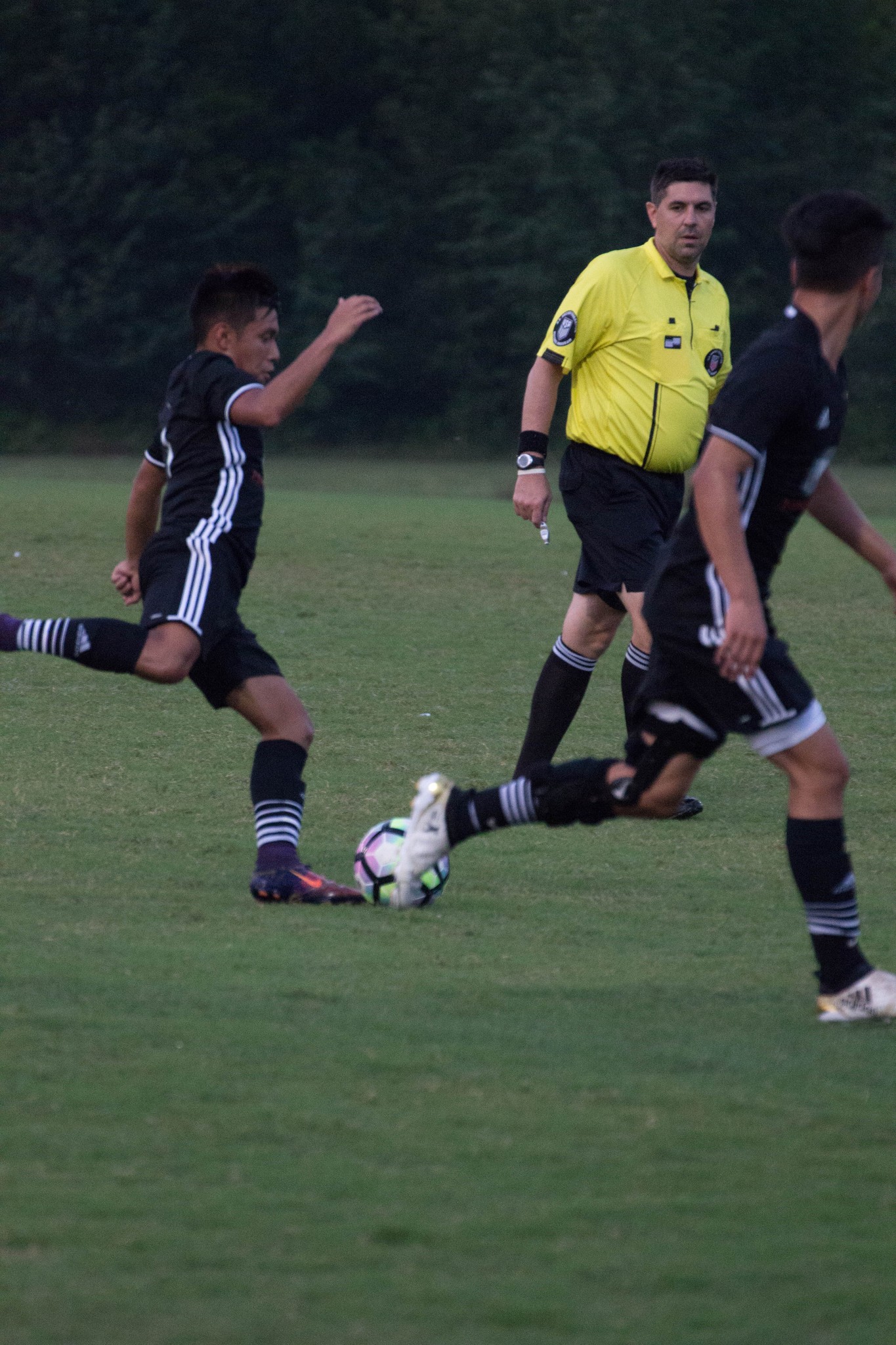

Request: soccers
left=354, top=816, right=452, bottom=911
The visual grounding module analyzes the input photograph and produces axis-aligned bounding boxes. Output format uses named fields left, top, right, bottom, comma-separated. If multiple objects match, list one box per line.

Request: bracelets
left=517, top=468, right=546, bottom=475
left=518, top=428, right=548, bottom=458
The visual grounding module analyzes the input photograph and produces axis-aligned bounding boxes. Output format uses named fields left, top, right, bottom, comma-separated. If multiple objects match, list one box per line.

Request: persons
left=394, top=190, right=896, bottom=1019
left=511, top=157, right=732, bottom=818
left=1, top=260, right=384, bottom=901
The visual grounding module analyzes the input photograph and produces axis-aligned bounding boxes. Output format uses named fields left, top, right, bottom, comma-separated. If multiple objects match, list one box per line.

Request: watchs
left=516, top=454, right=544, bottom=470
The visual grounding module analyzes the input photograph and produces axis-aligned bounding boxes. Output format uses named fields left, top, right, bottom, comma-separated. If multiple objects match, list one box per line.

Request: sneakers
left=389, top=770, right=458, bottom=908
left=249, top=863, right=364, bottom=904
left=817, top=970, right=896, bottom=1022
left=675, top=797, right=703, bottom=818
